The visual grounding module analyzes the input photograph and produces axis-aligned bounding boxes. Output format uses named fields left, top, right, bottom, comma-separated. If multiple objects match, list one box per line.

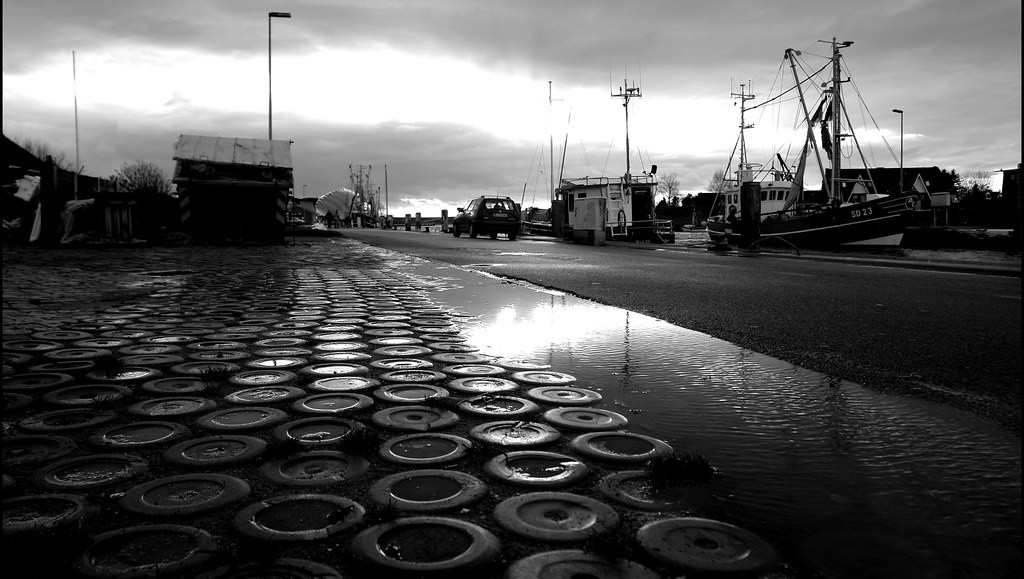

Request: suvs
left=452, top=195, right=522, bottom=241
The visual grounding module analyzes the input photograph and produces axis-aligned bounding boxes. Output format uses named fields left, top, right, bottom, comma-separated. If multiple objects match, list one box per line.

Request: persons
left=333, top=209, right=341, bottom=229
left=325, top=209, right=333, bottom=228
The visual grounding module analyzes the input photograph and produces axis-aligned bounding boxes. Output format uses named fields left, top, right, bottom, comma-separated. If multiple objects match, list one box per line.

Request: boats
left=766, top=48, right=904, bottom=248
left=719, top=77, right=796, bottom=224
left=550, top=55, right=675, bottom=244
left=704, top=36, right=925, bottom=246
left=520, top=80, right=564, bottom=235
